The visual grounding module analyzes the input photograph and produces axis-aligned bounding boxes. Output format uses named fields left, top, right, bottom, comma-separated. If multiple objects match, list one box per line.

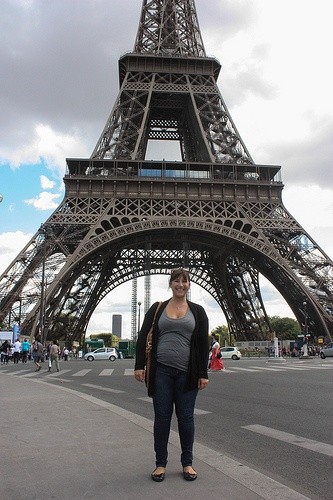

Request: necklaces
left=173, top=299, right=186, bottom=309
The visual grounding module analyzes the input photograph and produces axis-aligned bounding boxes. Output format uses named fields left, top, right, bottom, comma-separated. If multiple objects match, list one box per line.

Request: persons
left=0, top=338, right=79, bottom=364
left=133, top=268, right=210, bottom=482
left=207, top=333, right=216, bottom=369
left=268, top=335, right=332, bottom=358
left=46, top=340, right=60, bottom=372
left=209, top=336, right=224, bottom=372
left=32, top=338, right=43, bottom=372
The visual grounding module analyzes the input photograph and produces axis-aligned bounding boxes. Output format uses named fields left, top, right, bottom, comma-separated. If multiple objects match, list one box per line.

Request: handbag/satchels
left=48, top=357, right=52, bottom=368
left=144, top=300, right=162, bottom=386
left=216, top=352, right=222, bottom=358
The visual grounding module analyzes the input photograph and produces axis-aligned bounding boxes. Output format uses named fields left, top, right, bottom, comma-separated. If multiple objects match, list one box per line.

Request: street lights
left=39, top=223, right=52, bottom=341
left=303, top=302, right=308, bottom=356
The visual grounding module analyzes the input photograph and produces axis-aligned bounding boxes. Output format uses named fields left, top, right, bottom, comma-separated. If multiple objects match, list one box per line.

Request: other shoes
left=47, top=370, right=52, bottom=372
left=35, top=366, right=41, bottom=371
left=151, top=466, right=166, bottom=481
left=57, top=369, right=59, bottom=371
left=183, top=465, right=198, bottom=480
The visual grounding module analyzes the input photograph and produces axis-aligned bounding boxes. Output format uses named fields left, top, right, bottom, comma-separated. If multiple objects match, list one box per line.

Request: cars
left=220, top=347, right=242, bottom=360
left=84, top=348, right=119, bottom=361
left=320, top=342, right=333, bottom=359
